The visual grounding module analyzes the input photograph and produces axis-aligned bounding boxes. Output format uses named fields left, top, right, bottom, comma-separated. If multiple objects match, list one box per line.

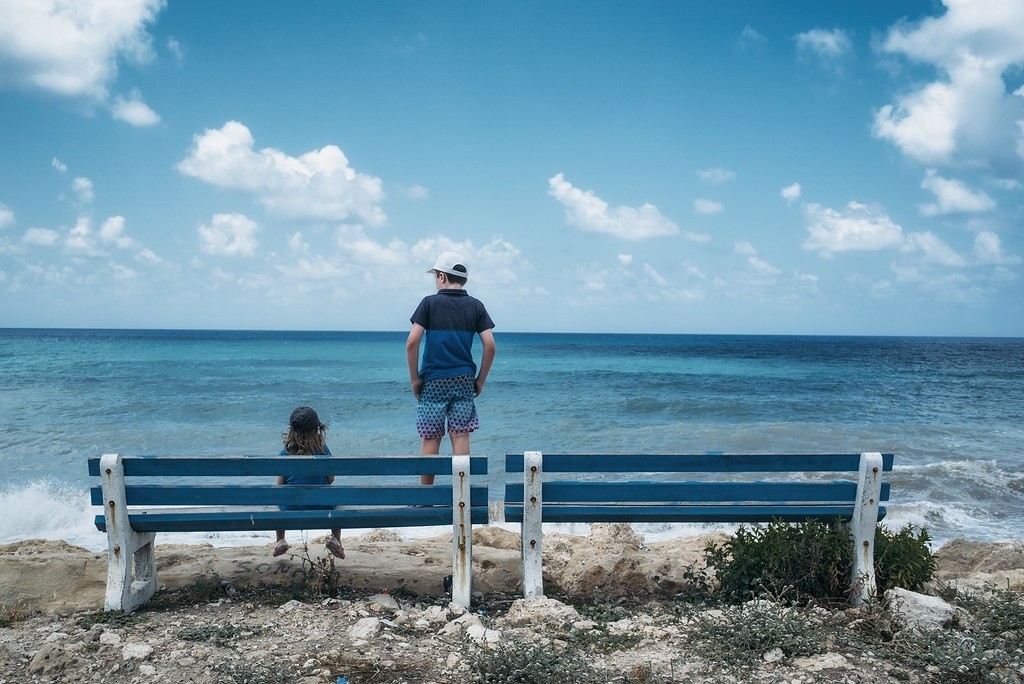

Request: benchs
left=505, top=451, right=895, bottom=607
left=88, top=453, right=488, bottom=615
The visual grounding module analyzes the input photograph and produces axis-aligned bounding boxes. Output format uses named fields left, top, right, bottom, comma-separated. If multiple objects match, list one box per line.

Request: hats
left=426, top=253, right=470, bottom=277
left=290, top=407, right=320, bottom=432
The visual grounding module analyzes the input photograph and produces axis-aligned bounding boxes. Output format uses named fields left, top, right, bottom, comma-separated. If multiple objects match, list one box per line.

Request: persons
left=273, top=406, right=346, bottom=559
left=406, top=252, right=497, bottom=512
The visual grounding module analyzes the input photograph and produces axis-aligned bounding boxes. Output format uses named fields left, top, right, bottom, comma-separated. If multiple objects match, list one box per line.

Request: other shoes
left=273, top=539, right=289, bottom=556
left=326, top=537, right=345, bottom=558
